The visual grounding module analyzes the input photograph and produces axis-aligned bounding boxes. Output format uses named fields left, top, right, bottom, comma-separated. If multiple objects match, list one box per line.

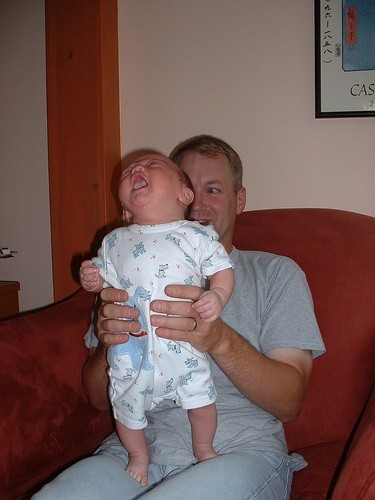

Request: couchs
left=0, top=208, right=375, bottom=500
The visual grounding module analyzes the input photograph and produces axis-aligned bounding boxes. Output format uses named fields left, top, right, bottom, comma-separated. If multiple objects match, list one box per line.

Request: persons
left=79, top=151, right=236, bottom=485
left=31, top=132, right=326, bottom=499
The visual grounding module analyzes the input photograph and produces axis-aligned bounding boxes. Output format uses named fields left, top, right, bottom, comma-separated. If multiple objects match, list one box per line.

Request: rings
left=187, top=316, right=199, bottom=332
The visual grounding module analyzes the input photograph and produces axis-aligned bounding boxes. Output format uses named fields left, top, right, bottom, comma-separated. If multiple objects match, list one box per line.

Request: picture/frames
left=314, top=0, right=375, bottom=118
